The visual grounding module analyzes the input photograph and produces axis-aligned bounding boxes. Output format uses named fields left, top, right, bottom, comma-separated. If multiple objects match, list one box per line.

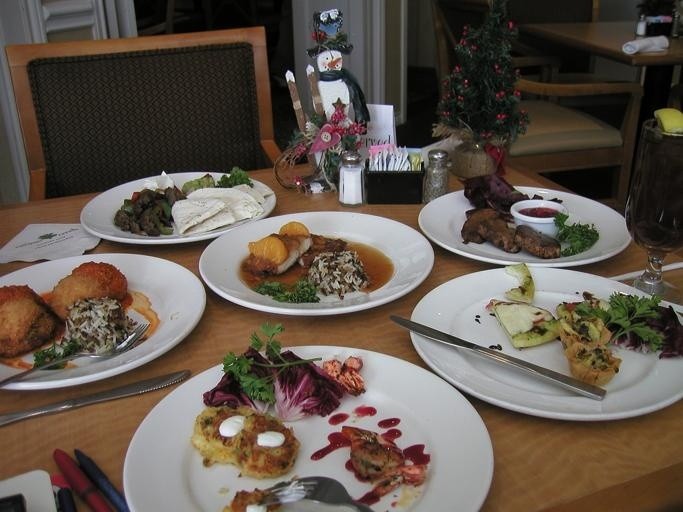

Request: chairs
left=429, top=0, right=645, bottom=217
left=5, top=24, right=291, bottom=203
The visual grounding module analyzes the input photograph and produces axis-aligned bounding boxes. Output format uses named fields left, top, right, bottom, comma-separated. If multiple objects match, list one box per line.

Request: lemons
left=655, top=108, right=683, bottom=135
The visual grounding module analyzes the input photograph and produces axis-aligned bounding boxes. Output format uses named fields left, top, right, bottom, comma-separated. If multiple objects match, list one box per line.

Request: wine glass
left=621, top=117, right=682, bottom=301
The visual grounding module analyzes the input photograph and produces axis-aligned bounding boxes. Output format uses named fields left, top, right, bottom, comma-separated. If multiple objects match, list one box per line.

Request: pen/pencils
left=58, top=486, right=73, bottom=512
left=54, top=448, right=111, bottom=512
left=74, top=446, right=129, bottom=511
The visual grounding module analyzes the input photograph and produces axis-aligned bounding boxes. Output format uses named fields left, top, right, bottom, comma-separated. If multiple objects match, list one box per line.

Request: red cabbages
left=205, top=345, right=346, bottom=421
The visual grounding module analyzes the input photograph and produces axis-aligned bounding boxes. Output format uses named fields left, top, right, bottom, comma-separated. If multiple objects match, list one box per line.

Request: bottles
left=634, top=14, right=647, bottom=37
left=669, top=11, right=680, bottom=38
left=337, top=152, right=366, bottom=207
left=421, top=149, right=448, bottom=202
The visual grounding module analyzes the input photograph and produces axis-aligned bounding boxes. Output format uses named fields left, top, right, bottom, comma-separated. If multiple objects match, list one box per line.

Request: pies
left=192, top=407, right=298, bottom=478
left=172, top=183, right=265, bottom=235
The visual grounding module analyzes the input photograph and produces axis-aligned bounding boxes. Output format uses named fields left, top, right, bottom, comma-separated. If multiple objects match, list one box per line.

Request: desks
left=520, top=19, right=682, bottom=66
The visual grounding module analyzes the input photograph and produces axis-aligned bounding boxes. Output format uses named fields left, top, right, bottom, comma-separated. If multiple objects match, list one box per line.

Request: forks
left=0, top=319, right=151, bottom=388
left=253, top=474, right=373, bottom=511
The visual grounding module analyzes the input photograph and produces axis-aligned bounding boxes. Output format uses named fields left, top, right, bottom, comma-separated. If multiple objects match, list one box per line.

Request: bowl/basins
left=510, top=198, right=570, bottom=239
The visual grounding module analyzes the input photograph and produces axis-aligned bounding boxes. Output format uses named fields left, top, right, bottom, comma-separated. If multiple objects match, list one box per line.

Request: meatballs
left=50, top=263, right=126, bottom=318
left=0, top=285, right=60, bottom=356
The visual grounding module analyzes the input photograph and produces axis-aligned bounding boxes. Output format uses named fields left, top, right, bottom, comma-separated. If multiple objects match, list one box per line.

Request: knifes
left=388, top=312, right=605, bottom=402
left=0, top=366, right=194, bottom=431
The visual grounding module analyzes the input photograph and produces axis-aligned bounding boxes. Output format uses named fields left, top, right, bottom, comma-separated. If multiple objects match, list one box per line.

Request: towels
left=622, top=35, right=671, bottom=56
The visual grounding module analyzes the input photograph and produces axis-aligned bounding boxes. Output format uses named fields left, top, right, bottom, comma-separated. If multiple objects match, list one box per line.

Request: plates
left=198, top=209, right=436, bottom=316
left=79, top=169, right=276, bottom=246
left=406, top=266, right=683, bottom=422
left=0, top=250, right=202, bottom=389
left=417, top=185, right=631, bottom=269
left=120, top=345, right=496, bottom=512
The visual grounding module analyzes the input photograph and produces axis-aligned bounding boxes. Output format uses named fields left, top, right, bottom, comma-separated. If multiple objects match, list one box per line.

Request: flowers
left=434, top=7, right=532, bottom=146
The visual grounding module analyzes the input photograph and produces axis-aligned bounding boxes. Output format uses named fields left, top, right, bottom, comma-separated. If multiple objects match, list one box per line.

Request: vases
left=449, top=137, right=498, bottom=184
